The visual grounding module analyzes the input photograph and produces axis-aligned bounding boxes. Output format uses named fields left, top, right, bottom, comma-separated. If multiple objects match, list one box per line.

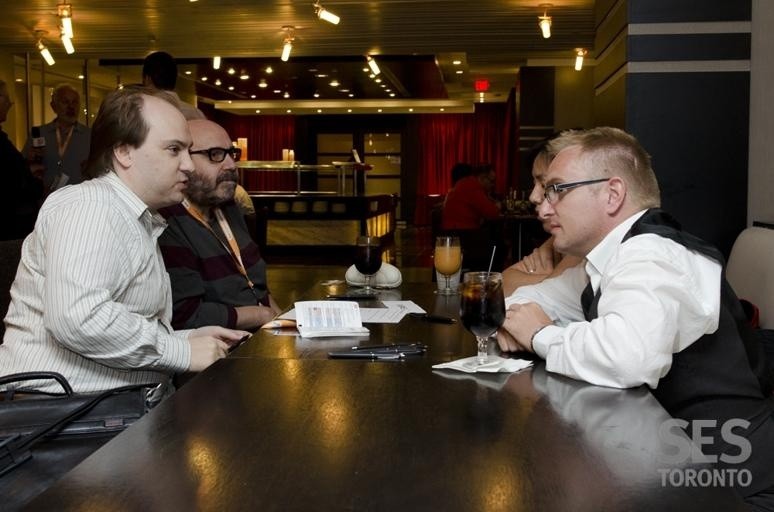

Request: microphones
left=31, top=126, right=46, bottom=162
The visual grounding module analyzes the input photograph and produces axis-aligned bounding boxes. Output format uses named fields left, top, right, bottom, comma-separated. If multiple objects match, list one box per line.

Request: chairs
left=0, top=239, right=23, bottom=343
left=723, top=223, right=774, bottom=337
left=431, top=203, right=441, bottom=283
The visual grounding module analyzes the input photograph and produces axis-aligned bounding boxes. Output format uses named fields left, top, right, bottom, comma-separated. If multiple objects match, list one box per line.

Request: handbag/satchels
left=0, top=371, right=163, bottom=512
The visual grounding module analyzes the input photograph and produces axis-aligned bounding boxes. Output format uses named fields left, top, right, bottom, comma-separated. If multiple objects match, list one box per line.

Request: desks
left=490, top=215, right=538, bottom=264
left=13, top=278, right=757, bottom=510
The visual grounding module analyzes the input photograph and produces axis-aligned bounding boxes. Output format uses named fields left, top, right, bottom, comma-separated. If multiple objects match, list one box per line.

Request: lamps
left=58, top=26, right=75, bottom=54
left=312, top=0, right=340, bottom=26
left=574, top=47, right=589, bottom=71
left=280, top=25, right=294, bottom=61
left=35, top=30, right=56, bottom=67
left=59, top=0, right=73, bottom=38
left=538, top=15, right=553, bottom=38
left=363, top=52, right=381, bottom=75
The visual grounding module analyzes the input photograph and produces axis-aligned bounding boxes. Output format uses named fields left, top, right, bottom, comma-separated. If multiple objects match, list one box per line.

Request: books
left=294, top=302, right=370, bottom=337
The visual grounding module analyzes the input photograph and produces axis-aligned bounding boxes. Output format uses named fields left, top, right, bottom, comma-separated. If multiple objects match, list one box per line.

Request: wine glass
left=355, top=236, right=382, bottom=295
left=434, top=236, right=462, bottom=295
left=460, top=272, right=506, bottom=369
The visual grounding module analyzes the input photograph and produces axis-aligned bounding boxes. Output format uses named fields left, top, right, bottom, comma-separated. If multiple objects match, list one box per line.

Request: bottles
left=288, top=150, right=294, bottom=161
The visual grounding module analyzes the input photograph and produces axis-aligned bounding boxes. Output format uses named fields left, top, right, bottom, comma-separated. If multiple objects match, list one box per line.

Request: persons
left=443, top=164, right=500, bottom=262
left=156, top=120, right=282, bottom=329
left=22, top=84, right=94, bottom=180
left=497, top=128, right=769, bottom=508
left=502, top=131, right=591, bottom=298
left=142, top=53, right=203, bottom=118
left=0, top=80, right=62, bottom=265
left=1, top=85, right=252, bottom=399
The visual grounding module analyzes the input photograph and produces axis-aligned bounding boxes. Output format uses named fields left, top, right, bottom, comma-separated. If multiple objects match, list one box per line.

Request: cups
left=237, top=138, right=247, bottom=161
left=283, top=149, right=288, bottom=161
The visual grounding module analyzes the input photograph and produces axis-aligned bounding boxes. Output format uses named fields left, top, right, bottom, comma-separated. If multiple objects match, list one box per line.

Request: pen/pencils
left=327, top=341, right=429, bottom=359
left=429, top=316, right=458, bottom=323
left=227, top=334, right=250, bottom=353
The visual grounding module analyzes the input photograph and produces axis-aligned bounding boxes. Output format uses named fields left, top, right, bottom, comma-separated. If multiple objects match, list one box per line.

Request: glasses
left=189, top=147, right=241, bottom=162
left=544, top=179, right=610, bottom=202
